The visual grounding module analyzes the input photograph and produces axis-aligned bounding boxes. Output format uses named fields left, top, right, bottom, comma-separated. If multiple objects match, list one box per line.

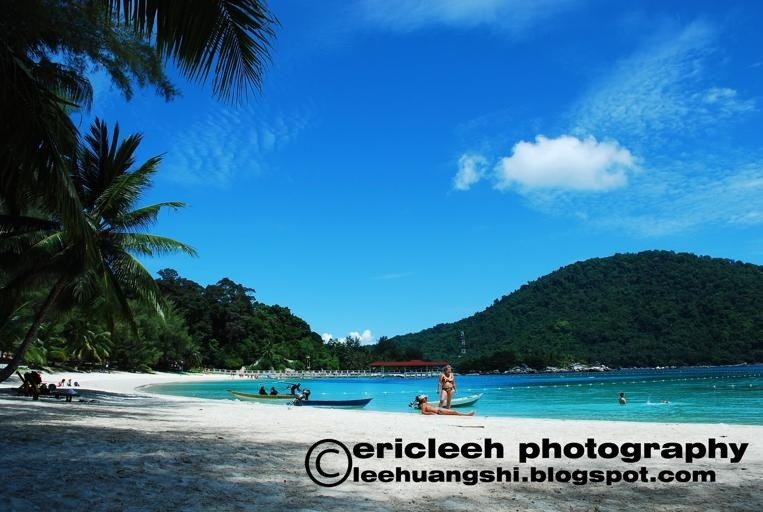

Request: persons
left=299, top=390, right=310, bottom=399
left=291, top=382, right=302, bottom=395
left=59, top=378, right=81, bottom=388
left=417, top=396, right=475, bottom=416
left=269, top=387, right=278, bottom=394
left=258, top=386, right=267, bottom=394
left=438, top=365, right=456, bottom=409
left=619, top=391, right=626, bottom=406
left=67, top=389, right=74, bottom=402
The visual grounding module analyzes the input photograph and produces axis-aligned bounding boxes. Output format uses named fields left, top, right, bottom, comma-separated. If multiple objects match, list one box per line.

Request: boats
left=294, top=396, right=374, bottom=406
left=411, top=392, right=485, bottom=408
left=232, top=391, right=299, bottom=400
